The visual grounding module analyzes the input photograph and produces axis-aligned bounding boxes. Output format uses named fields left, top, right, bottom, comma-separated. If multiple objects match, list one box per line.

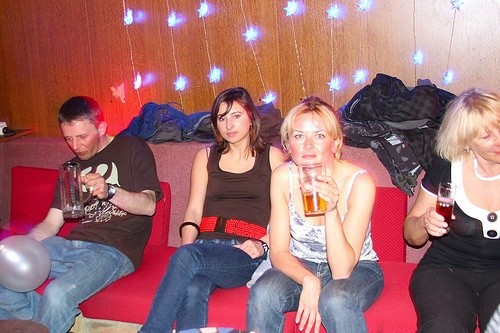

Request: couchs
left=0, top=134, right=480, bottom=333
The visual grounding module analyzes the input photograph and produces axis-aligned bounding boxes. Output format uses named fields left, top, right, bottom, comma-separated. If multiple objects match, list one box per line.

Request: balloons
left=0, top=235, right=51, bottom=293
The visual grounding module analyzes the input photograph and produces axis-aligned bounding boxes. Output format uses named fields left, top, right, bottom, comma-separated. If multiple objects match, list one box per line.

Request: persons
left=0, top=97, right=165, bottom=333
left=137, top=88, right=286, bottom=333
left=403, top=88, right=500, bottom=333
left=247, top=96, right=383, bottom=333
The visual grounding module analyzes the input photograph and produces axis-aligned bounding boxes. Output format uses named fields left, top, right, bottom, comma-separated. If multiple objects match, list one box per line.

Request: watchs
left=100, top=183, right=116, bottom=202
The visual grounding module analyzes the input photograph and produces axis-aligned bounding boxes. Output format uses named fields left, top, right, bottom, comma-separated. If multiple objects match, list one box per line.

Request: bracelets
left=256, top=240, right=269, bottom=254
left=179, top=222, right=201, bottom=239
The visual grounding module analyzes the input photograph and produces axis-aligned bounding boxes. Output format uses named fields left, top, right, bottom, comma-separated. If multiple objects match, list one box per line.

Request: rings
left=425, top=223, right=430, bottom=227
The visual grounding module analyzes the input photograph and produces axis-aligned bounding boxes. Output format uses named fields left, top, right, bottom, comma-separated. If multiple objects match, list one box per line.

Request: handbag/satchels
left=334, top=103, right=424, bottom=195
left=153, top=121, right=183, bottom=144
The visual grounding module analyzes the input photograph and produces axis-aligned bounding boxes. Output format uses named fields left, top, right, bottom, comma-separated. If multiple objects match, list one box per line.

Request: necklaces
left=473, top=158, right=500, bottom=181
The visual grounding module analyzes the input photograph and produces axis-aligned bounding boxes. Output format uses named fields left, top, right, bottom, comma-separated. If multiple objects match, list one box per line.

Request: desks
left=2, top=319, right=50, bottom=333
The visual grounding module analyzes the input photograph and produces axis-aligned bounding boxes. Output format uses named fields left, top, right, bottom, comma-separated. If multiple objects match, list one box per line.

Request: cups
left=297, top=162, right=329, bottom=217
left=57, top=161, right=93, bottom=218
left=436, top=181, right=457, bottom=231
